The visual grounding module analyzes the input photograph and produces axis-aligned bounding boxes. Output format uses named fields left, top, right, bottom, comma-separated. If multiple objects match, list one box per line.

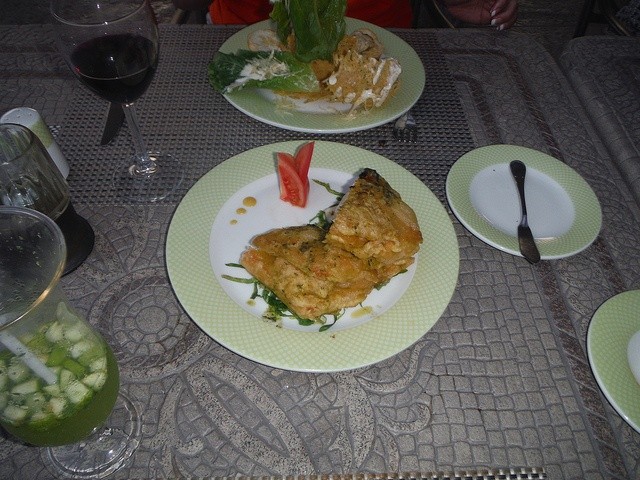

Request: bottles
left=0, top=107, right=70, bottom=180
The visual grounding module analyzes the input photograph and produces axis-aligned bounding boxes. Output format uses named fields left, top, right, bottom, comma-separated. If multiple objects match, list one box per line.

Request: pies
left=239, top=168, right=424, bottom=321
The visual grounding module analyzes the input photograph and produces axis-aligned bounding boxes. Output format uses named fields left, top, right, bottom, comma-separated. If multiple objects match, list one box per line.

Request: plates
left=208, top=16, right=428, bottom=136
left=162, top=138, right=464, bottom=376
left=445, top=144, right=603, bottom=259
left=587, top=289, right=639, bottom=431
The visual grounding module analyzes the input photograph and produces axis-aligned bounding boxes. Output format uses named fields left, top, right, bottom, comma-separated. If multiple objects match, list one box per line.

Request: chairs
left=571, top=0, right=640, bottom=37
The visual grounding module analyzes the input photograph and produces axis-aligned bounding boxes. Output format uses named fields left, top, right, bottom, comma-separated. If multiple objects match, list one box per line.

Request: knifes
left=100, top=101, right=127, bottom=147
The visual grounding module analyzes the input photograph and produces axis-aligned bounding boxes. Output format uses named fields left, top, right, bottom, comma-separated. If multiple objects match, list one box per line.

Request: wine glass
left=47, top=0, right=183, bottom=202
left=0, top=206, right=141, bottom=479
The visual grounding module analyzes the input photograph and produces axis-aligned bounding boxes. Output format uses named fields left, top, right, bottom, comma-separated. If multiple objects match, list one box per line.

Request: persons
left=170, top=0, right=521, bottom=32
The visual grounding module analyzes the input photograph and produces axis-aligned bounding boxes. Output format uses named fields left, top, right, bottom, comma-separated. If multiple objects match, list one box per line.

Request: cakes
left=269, top=32, right=403, bottom=115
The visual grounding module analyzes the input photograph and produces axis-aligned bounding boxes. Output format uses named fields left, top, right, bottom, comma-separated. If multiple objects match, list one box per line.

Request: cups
left=0, top=122, right=94, bottom=277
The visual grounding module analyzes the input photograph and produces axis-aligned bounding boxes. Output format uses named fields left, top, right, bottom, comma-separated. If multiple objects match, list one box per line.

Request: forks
left=393, top=111, right=418, bottom=143
